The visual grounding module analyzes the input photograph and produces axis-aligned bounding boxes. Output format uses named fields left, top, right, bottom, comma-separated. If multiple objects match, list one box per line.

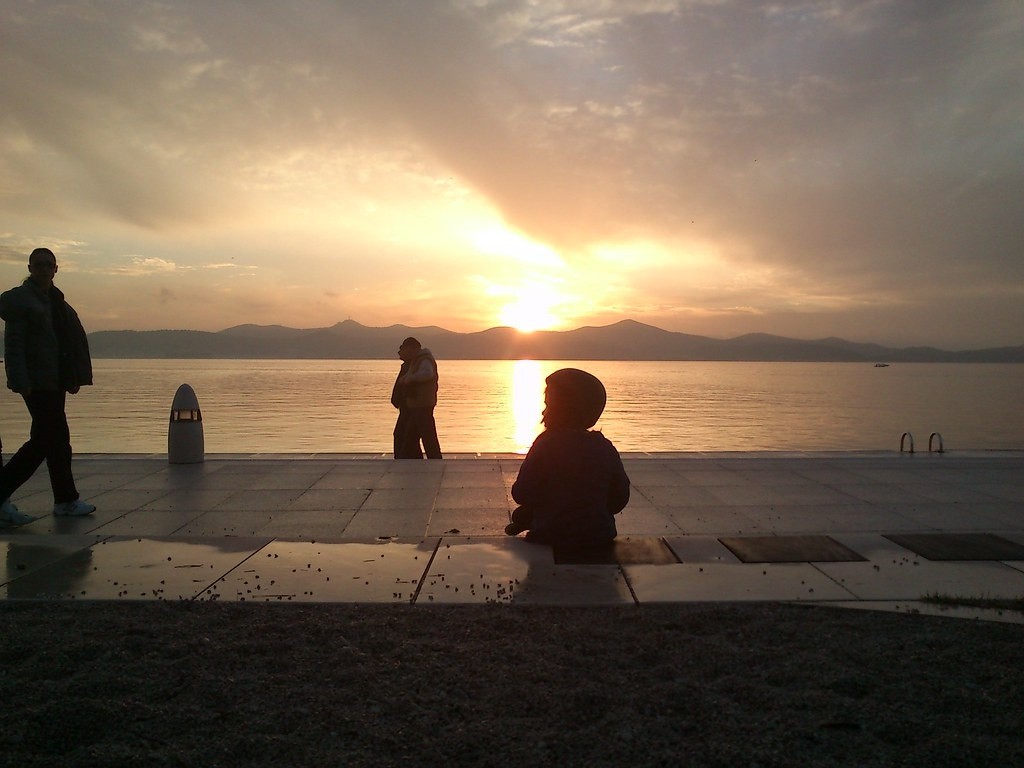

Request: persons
left=0, top=248, right=97, bottom=527
left=505, top=368, right=631, bottom=549
left=391, top=337, right=442, bottom=459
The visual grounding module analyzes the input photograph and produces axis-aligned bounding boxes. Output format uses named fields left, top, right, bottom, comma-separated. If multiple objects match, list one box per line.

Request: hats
left=544, top=367, right=607, bottom=421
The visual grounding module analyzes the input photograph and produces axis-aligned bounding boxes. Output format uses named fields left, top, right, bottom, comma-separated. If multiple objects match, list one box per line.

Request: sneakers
left=0, top=499, right=37, bottom=525
left=53, top=496, right=96, bottom=516
